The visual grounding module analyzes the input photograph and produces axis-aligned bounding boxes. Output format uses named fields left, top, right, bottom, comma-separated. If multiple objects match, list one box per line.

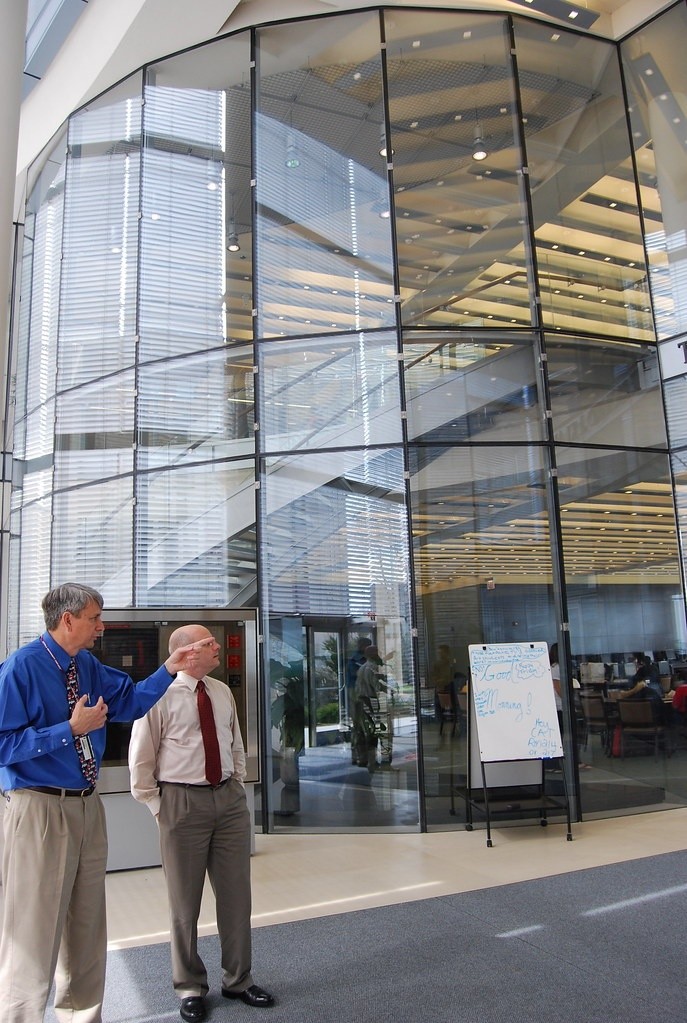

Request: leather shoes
left=180, top=997, right=206, bottom=1021
left=221, top=984, right=274, bottom=1007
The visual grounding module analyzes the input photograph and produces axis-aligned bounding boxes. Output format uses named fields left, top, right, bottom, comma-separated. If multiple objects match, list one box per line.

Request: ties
left=198, top=681, right=222, bottom=788
left=67, top=658, right=96, bottom=787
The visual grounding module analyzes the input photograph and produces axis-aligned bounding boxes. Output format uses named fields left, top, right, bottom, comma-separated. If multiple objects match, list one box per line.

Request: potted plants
left=270, top=660, right=305, bottom=787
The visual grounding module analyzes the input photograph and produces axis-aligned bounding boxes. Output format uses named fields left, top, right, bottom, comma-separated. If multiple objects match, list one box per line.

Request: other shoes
left=379, top=765, right=399, bottom=772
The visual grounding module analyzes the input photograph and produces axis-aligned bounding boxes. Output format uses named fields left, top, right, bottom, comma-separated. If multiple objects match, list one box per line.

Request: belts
left=23, top=786, right=95, bottom=799
left=181, top=779, right=228, bottom=789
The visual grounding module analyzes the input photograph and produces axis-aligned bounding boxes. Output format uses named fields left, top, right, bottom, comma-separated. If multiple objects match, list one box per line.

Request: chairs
left=577, top=693, right=673, bottom=763
left=659, top=676, right=672, bottom=691
left=437, top=691, right=468, bottom=737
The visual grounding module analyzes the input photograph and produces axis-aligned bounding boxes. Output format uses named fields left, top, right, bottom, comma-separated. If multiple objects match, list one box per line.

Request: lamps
left=206, top=140, right=219, bottom=188
left=472, top=92, right=489, bottom=160
left=284, top=97, right=300, bottom=168
left=380, top=190, right=391, bottom=217
left=379, top=88, right=394, bottom=157
left=110, top=225, right=123, bottom=254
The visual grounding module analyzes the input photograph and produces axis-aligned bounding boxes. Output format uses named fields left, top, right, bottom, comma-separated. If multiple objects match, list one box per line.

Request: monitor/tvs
left=572, top=648, right=677, bottom=679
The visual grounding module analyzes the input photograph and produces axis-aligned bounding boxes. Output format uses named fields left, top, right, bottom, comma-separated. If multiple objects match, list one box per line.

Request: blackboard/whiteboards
left=465, top=642, right=565, bottom=790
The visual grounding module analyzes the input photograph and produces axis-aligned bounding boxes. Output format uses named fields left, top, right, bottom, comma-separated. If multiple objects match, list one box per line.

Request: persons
left=662, top=655, right=669, bottom=663
left=663, top=671, right=687, bottom=725
left=346, top=637, right=401, bottom=774
left=544, top=643, right=592, bottom=773
left=601, top=677, right=646, bottom=758
left=628, top=657, right=639, bottom=672
left=632, top=655, right=660, bottom=688
left=0, top=582, right=216, bottom=1023
left=129, top=624, right=275, bottom=1022
left=427, top=643, right=461, bottom=739
left=682, top=654, right=687, bottom=663
left=593, top=655, right=614, bottom=685
